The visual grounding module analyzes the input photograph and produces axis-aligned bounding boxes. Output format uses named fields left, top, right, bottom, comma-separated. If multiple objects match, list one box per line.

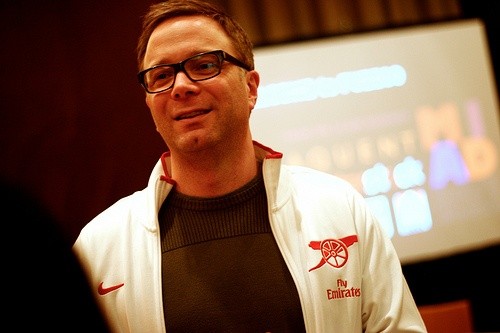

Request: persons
left=73, top=0, right=427, bottom=333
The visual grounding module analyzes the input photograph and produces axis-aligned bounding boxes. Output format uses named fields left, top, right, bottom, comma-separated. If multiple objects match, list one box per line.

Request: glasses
left=134, top=48, right=254, bottom=95
